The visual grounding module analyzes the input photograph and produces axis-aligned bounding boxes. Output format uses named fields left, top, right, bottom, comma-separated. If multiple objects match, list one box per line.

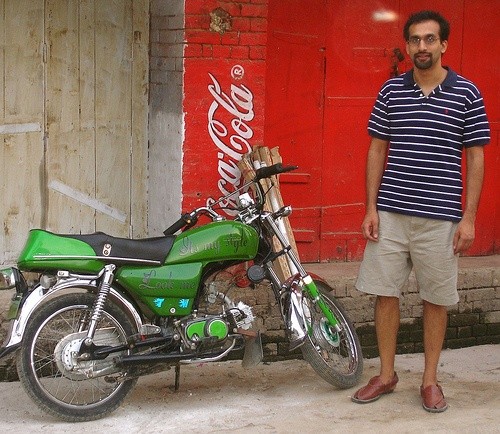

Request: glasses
left=407, top=36, right=442, bottom=46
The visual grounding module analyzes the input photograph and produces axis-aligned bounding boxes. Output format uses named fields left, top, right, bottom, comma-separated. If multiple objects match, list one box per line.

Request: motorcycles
left=0, top=162, right=365, bottom=423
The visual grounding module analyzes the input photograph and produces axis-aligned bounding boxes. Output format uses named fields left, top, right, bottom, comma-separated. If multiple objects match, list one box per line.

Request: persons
left=351, top=10, right=491, bottom=413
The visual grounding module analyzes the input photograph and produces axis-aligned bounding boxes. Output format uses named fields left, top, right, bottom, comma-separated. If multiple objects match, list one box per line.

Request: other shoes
left=420, top=384, right=448, bottom=412
left=351, top=370, right=399, bottom=403
